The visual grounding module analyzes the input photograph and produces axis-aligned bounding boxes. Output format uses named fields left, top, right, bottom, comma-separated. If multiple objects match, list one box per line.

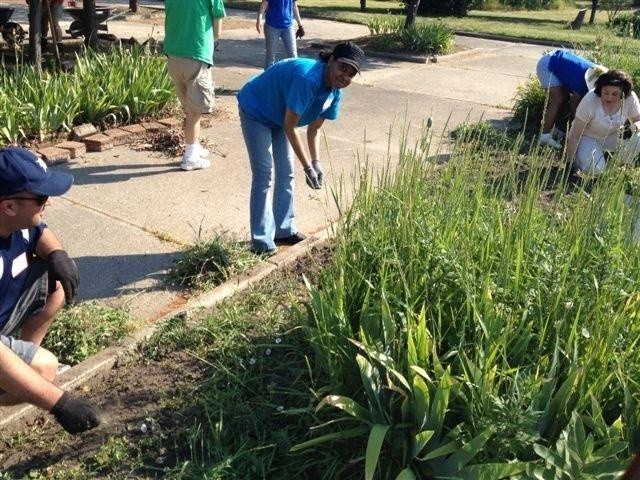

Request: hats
left=332, top=42, right=364, bottom=74
left=1, top=148, right=73, bottom=196
left=585, top=64, right=607, bottom=91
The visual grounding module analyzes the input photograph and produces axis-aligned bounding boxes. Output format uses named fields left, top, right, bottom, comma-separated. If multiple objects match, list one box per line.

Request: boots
left=41, top=37, right=63, bottom=57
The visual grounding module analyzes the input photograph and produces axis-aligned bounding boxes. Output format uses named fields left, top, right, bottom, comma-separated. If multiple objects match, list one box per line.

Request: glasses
left=2, top=194, right=48, bottom=207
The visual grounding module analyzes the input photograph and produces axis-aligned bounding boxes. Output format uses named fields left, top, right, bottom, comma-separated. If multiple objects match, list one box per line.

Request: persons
left=1, top=148, right=99, bottom=435
left=236, top=43, right=364, bottom=258
left=256, top=0, right=306, bottom=71
left=563, top=69, right=640, bottom=179
left=163, top=1, right=227, bottom=170
left=536, top=49, right=608, bottom=148
left=40, top=0, right=65, bottom=54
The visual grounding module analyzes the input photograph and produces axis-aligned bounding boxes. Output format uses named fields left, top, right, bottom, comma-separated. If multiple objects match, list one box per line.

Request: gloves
left=47, top=250, right=81, bottom=305
left=619, top=124, right=638, bottom=139
left=296, top=26, right=305, bottom=39
left=48, top=391, right=101, bottom=436
left=303, top=160, right=322, bottom=190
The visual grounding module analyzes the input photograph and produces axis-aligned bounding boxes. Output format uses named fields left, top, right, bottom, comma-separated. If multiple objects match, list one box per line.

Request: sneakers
left=180, top=141, right=211, bottom=170
left=539, top=127, right=566, bottom=150
left=250, top=232, right=306, bottom=256
